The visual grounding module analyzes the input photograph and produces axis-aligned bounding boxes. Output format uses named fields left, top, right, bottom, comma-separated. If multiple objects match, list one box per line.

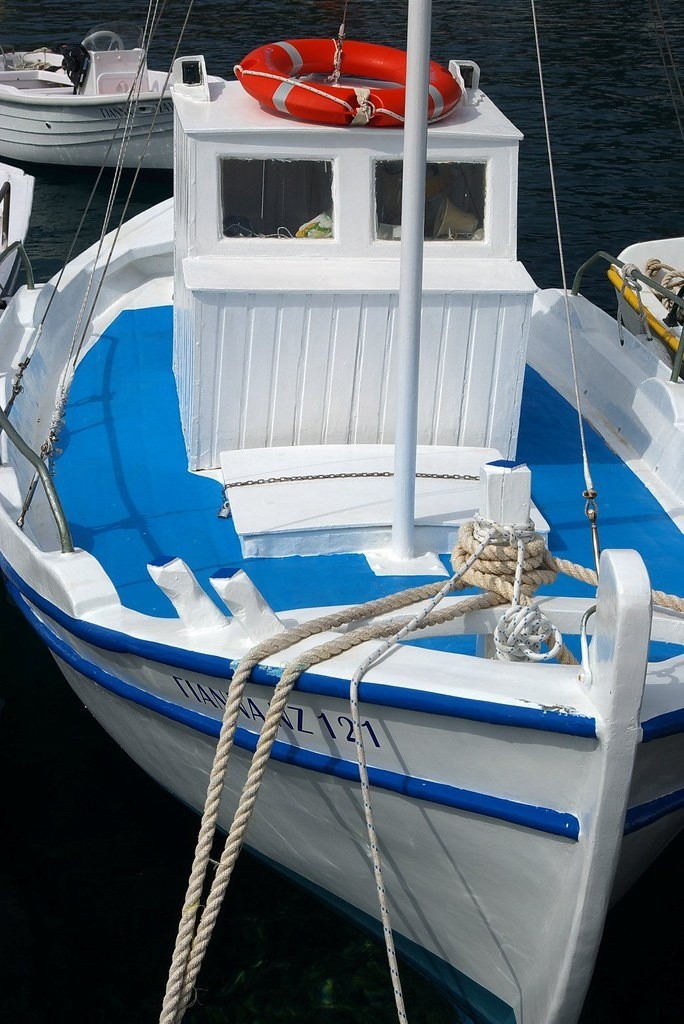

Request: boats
left=607, top=237, right=684, bottom=360
left=0, top=0, right=684, bottom=1024
left=0, top=31, right=174, bottom=169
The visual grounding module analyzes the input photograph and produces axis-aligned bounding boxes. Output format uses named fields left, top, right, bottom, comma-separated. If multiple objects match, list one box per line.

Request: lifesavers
left=234, top=39, right=462, bottom=126
